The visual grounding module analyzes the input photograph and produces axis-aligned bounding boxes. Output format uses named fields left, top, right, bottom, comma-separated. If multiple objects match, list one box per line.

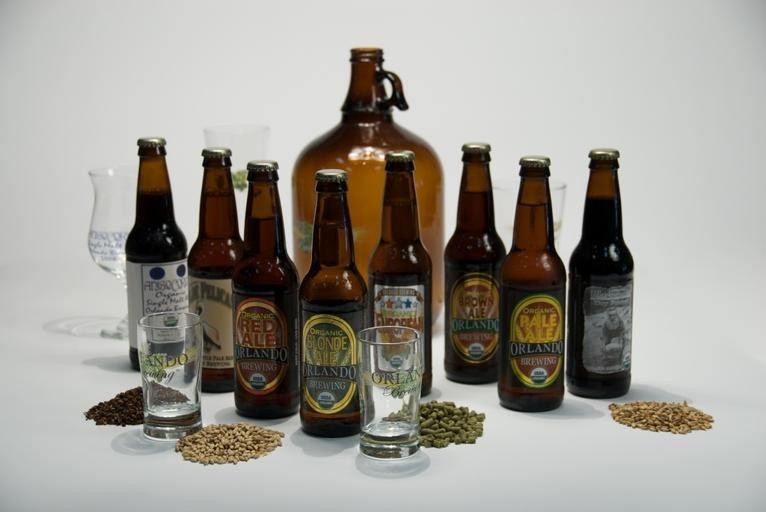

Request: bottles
left=296, top=169, right=374, bottom=438
left=444, top=141, right=507, bottom=384
left=367, top=149, right=432, bottom=397
left=125, top=138, right=189, bottom=372
left=186, top=147, right=245, bottom=394
left=497, top=155, right=567, bottom=412
left=231, top=159, right=301, bottom=420
left=566, top=149, right=635, bottom=399
left=291, top=48, right=444, bottom=329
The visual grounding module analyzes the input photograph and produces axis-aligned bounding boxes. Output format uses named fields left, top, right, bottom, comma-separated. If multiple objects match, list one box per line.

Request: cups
left=136, top=311, right=204, bottom=442
left=355, top=325, right=422, bottom=461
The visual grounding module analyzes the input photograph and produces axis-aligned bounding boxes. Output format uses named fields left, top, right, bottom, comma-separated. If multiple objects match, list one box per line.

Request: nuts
left=608, top=400, right=714, bottom=435
left=174, top=424, right=285, bottom=465
left=383, top=401, right=485, bottom=448
left=85, top=380, right=188, bottom=427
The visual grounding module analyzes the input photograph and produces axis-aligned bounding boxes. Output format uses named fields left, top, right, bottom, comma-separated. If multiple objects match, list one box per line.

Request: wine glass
left=87, top=167, right=137, bottom=340
